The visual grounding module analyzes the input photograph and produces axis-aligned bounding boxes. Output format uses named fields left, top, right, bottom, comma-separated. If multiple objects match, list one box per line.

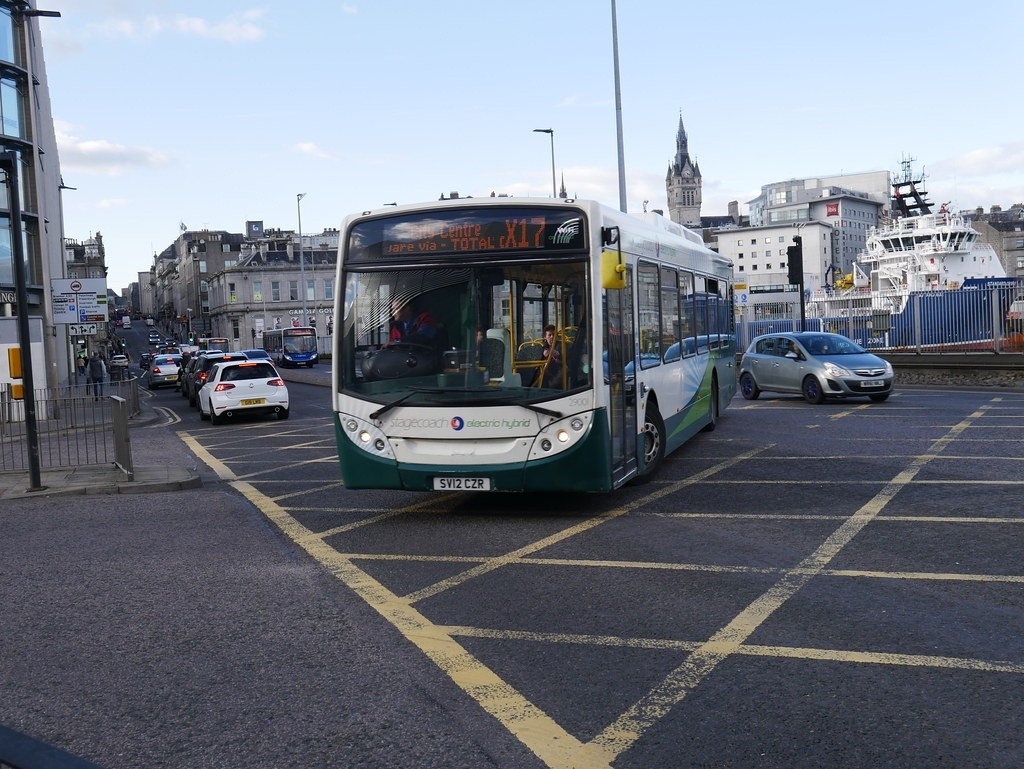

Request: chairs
left=478, top=325, right=579, bottom=387
left=764, top=342, right=774, bottom=354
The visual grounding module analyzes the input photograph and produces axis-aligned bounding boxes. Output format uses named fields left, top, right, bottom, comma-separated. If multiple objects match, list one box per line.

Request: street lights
left=533, top=128, right=556, bottom=198
left=297, top=192, right=308, bottom=328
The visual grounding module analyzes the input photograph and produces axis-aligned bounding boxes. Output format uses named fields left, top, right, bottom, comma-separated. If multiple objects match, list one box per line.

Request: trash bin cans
left=110, top=365, right=122, bottom=386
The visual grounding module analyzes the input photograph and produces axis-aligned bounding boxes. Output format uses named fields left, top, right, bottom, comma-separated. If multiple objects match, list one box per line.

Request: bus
left=330, top=196, right=738, bottom=501
left=114, top=308, right=127, bottom=326
left=263, top=327, right=319, bottom=368
left=196, top=338, right=229, bottom=353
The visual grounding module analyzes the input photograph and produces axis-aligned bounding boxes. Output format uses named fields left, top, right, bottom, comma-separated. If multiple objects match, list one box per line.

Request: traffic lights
left=121, top=338, right=125, bottom=347
left=251, top=330, right=256, bottom=338
left=189, top=332, right=193, bottom=342
left=786, top=246, right=800, bottom=285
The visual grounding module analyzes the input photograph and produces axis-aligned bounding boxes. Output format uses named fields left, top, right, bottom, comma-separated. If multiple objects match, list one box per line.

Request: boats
left=734, top=211, right=1024, bottom=348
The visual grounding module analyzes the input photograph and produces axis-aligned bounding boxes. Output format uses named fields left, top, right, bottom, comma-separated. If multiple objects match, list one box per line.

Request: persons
left=383, top=290, right=438, bottom=352
left=474, top=327, right=484, bottom=361
left=535, top=325, right=568, bottom=384
left=78, top=351, right=107, bottom=401
left=790, top=341, right=805, bottom=360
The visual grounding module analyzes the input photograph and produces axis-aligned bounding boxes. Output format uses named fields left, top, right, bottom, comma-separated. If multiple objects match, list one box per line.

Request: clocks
left=684, top=169, right=691, bottom=176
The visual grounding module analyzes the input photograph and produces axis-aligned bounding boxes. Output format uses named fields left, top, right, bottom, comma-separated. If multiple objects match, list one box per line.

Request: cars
left=111, top=355, right=128, bottom=368
left=738, top=330, right=895, bottom=405
left=140, top=329, right=290, bottom=425
left=122, top=322, right=132, bottom=329
